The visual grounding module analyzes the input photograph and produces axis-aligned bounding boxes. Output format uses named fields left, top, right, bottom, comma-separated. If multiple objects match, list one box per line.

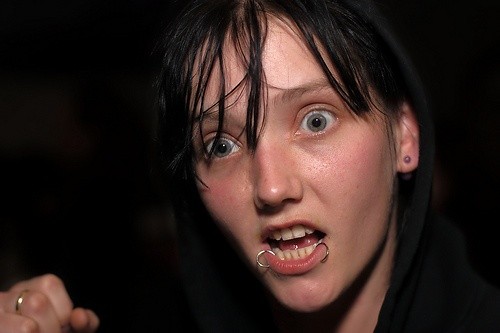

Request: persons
left=0, top=0, right=500, bottom=333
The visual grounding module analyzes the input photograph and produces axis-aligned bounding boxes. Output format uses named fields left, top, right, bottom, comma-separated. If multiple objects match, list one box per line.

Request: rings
left=15, top=290, right=30, bottom=315
